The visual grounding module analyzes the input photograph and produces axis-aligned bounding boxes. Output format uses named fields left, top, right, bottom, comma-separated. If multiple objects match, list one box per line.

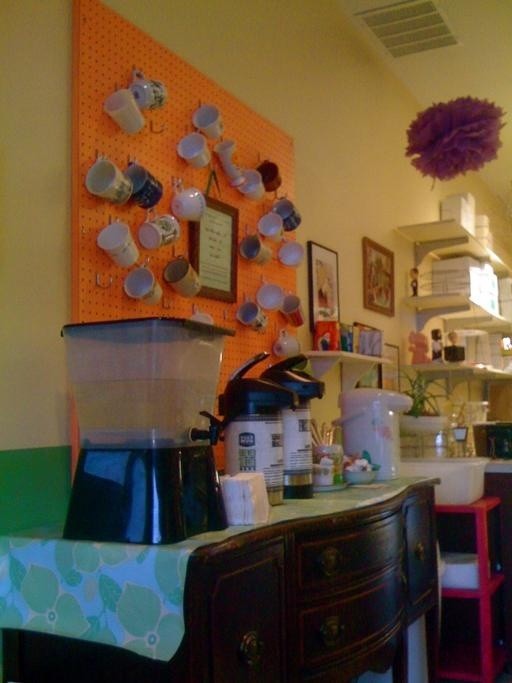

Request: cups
left=255, top=157, right=282, bottom=191
left=272, top=196, right=303, bottom=232
left=256, top=276, right=283, bottom=311
left=139, top=207, right=181, bottom=251
left=86, top=153, right=134, bottom=207
left=237, top=296, right=267, bottom=332
left=259, top=212, right=285, bottom=244
left=236, top=169, right=265, bottom=199
left=190, top=311, right=217, bottom=327
left=279, top=294, right=306, bottom=328
left=128, top=70, right=168, bottom=110
left=239, top=232, right=272, bottom=265
left=123, top=264, right=163, bottom=307
left=214, top=137, right=245, bottom=185
left=104, top=88, right=147, bottom=134
left=169, top=177, right=207, bottom=221
left=190, top=103, right=224, bottom=137
left=176, top=129, right=211, bottom=168
left=313, top=445, right=342, bottom=492
left=164, top=255, right=202, bottom=299
left=276, top=236, right=304, bottom=266
left=273, top=328, right=301, bottom=358
left=122, top=162, right=163, bottom=208
left=95, top=221, right=138, bottom=267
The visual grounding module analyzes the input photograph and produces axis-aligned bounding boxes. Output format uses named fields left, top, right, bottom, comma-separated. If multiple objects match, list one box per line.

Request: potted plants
left=396, top=368, right=455, bottom=458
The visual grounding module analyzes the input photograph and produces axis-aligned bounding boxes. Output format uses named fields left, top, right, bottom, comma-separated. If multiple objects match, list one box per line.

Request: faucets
left=189, top=409, right=224, bottom=446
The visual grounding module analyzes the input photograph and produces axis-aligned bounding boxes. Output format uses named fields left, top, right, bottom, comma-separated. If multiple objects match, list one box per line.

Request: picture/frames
left=361, top=237, right=395, bottom=318
left=187, top=193, right=239, bottom=303
left=307, top=241, right=341, bottom=333
left=454, top=426, right=469, bottom=441
left=382, top=343, right=400, bottom=393
left=354, top=321, right=382, bottom=389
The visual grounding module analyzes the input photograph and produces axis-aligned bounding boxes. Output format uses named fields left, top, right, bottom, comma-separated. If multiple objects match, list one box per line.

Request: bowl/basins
left=342, top=470, right=375, bottom=486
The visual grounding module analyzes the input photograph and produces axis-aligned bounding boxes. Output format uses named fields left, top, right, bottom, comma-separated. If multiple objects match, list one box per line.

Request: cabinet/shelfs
left=0, top=476, right=442, bottom=682
left=434, top=495, right=509, bottom=683
left=398, top=219, right=512, bottom=379
